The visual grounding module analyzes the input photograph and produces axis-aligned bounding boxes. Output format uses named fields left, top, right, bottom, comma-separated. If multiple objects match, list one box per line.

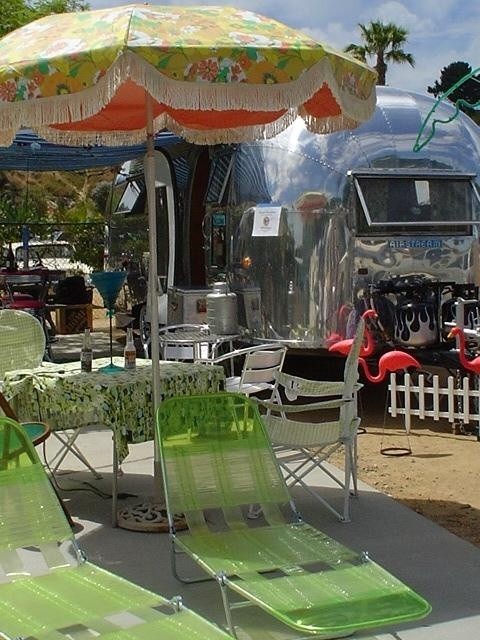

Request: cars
left=3, top=241, right=95, bottom=304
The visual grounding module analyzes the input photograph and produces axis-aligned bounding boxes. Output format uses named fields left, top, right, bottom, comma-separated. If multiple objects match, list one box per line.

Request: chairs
left=0, top=416, right=235, bottom=640
left=144, top=324, right=221, bottom=366
left=154, top=392, right=433, bottom=640
left=194, top=343, right=287, bottom=440
left=246, top=320, right=366, bottom=523
left=0, top=310, right=47, bottom=375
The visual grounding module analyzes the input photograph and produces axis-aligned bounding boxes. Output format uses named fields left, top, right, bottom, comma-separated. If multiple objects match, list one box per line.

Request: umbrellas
left=0, top=1, right=380, bottom=508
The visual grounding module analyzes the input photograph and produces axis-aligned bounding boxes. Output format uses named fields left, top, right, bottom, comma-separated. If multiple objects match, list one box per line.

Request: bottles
left=80, top=328, right=93, bottom=372
left=123, top=327, right=136, bottom=370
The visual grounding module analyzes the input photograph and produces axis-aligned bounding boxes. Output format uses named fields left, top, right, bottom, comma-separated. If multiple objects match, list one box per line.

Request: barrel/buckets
left=287, top=281, right=304, bottom=324
left=205, top=282, right=238, bottom=334
